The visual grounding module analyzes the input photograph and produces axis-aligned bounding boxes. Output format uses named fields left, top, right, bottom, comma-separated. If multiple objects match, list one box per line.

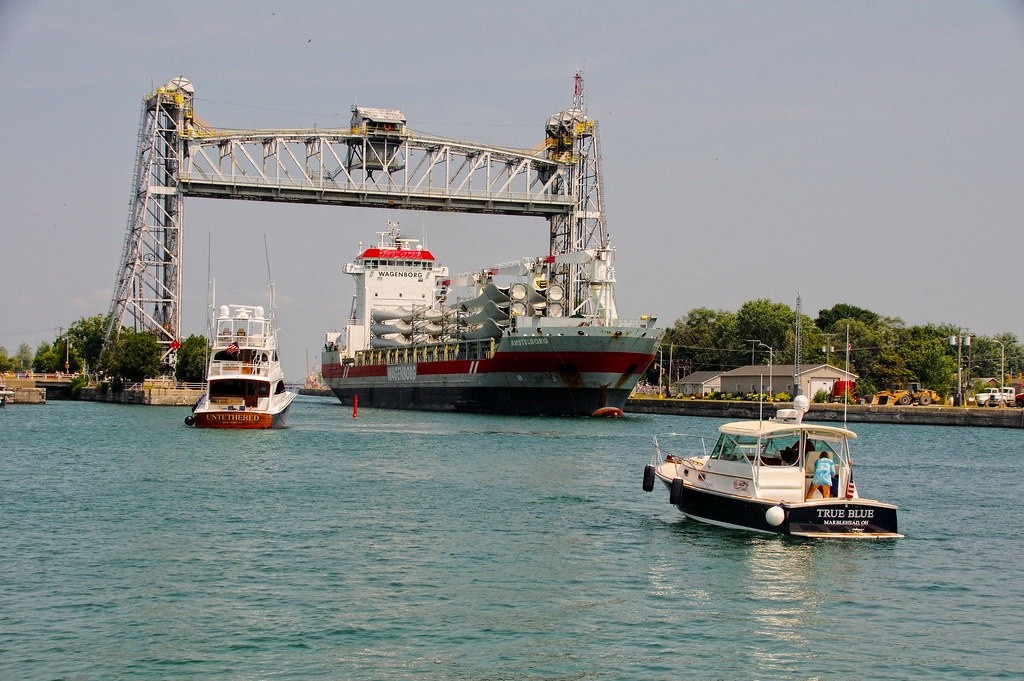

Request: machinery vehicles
left=870, top=382, right=940, bottom=407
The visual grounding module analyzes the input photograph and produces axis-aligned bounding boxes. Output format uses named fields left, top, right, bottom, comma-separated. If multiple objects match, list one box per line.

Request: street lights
left=758, top=343, right=772, bottom=399
left=949, top=326, right=971, bottom=404
left=990, top=340, right=1005, bottom=406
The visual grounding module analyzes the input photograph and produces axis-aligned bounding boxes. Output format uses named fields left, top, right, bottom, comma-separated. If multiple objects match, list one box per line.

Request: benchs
left=805, top=451, right=833, bottom=477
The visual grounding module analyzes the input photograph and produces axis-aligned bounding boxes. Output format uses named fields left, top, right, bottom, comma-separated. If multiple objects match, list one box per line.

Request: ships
left=319, top=217, right=668, bottom=418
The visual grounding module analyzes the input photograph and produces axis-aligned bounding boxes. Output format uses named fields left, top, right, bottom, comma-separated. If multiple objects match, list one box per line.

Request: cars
left=1015, top=393, right=1024, bottom=408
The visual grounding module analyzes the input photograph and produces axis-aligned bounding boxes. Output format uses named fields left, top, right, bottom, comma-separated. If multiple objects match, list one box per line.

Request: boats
left=184, top=302, right=299, bottom=429
left=641, top=390, right=907, bottom=543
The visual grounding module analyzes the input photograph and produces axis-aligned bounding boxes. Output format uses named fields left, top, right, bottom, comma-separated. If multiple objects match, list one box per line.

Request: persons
left=781, top=429, right=816, bottom=466
left=806, top=450, right=837, bottom=498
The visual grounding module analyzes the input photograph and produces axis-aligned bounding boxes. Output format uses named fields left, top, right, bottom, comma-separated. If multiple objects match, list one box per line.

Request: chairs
left=223, top=328, right=231, bottom=335
left=237, top=329, right=245, bottom=335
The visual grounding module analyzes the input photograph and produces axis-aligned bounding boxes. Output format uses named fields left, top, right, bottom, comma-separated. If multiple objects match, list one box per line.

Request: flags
left=226, top=341, right=239, bottom=353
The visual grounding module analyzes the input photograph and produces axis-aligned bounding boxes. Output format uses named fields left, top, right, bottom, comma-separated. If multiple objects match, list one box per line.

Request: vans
left=974, top=386, right=1016, bottom=406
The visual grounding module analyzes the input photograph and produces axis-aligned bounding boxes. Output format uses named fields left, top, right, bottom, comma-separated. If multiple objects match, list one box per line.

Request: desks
left=779, top=449, right=798, bottom=465
